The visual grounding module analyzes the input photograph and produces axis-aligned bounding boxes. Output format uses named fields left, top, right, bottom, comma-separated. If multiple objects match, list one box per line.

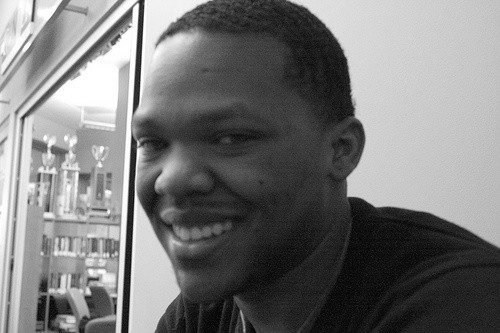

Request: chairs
left=65, top=284, right=116, bottom=333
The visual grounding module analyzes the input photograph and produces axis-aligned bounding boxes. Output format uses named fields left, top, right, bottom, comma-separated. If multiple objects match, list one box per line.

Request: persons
left=131, top=0, right=500, bottom=333
